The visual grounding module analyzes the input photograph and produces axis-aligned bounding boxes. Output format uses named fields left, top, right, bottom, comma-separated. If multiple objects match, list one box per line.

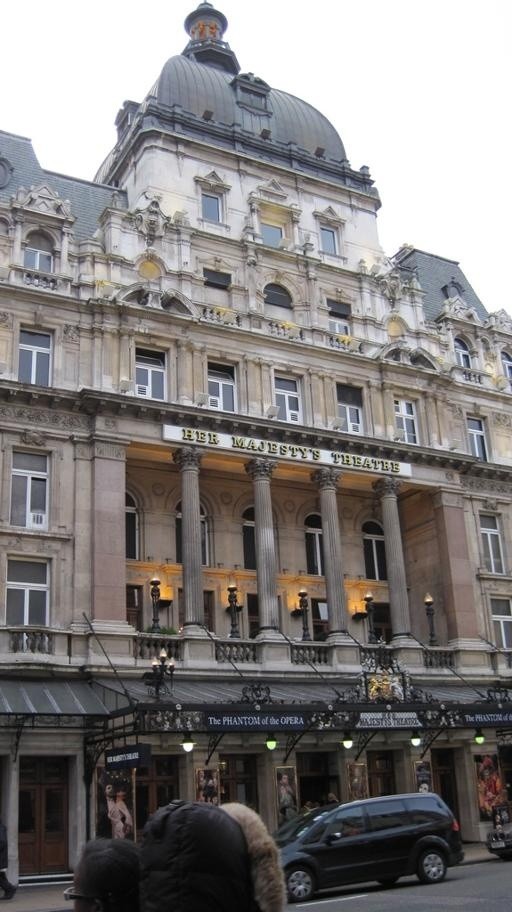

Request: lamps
left=265, top=731, right=278, bottom=751
left=392, top=427, right=406, bottom=442
left=474, top=728, right=485, bottom=745
left=329, top=416, right=347, bottom=430
left=180, top=733, right=197, bottom=752
left=410, top=730, right=422, bottom=747
left=120, top=379, right=137, bottom=395
left=342, top=731, right=353, bottom=749
left=193, top=392, right=211, bottom=409
left=265, top=404, right=282, bottom=419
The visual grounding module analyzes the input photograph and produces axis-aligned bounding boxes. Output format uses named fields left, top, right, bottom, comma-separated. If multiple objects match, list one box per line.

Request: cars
left=485, top=820, right=512, bottom=862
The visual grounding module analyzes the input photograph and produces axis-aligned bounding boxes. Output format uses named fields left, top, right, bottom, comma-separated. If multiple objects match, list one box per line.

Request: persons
left=97, top=772, right=133, bottom=839
left=0, top=817, right=16, bottom=899
left=477, top=756, right=508, bottom=818
left=279, top=773, right=295, bottom=824
left=198, top=770, right=217, bottom=804
left=74, top=798, right=287, bottom=912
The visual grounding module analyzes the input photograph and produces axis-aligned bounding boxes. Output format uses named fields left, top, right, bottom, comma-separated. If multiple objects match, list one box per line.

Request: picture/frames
left=196, top=767, right=221, bottom=806
left=274, top=766, right=301, bottom=828
left=471, top=751, right=507, bottom=820
left=346, top=762, right=369, bottom=799
left=413, top=759, right=435, bottom=794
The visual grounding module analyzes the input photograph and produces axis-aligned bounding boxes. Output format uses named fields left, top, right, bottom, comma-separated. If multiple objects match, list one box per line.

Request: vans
left=269, top=790, right=466, bottom=904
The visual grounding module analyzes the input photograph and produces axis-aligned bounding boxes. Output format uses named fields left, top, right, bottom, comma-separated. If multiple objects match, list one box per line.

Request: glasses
left=63, top=886, right=96, bottom=902
